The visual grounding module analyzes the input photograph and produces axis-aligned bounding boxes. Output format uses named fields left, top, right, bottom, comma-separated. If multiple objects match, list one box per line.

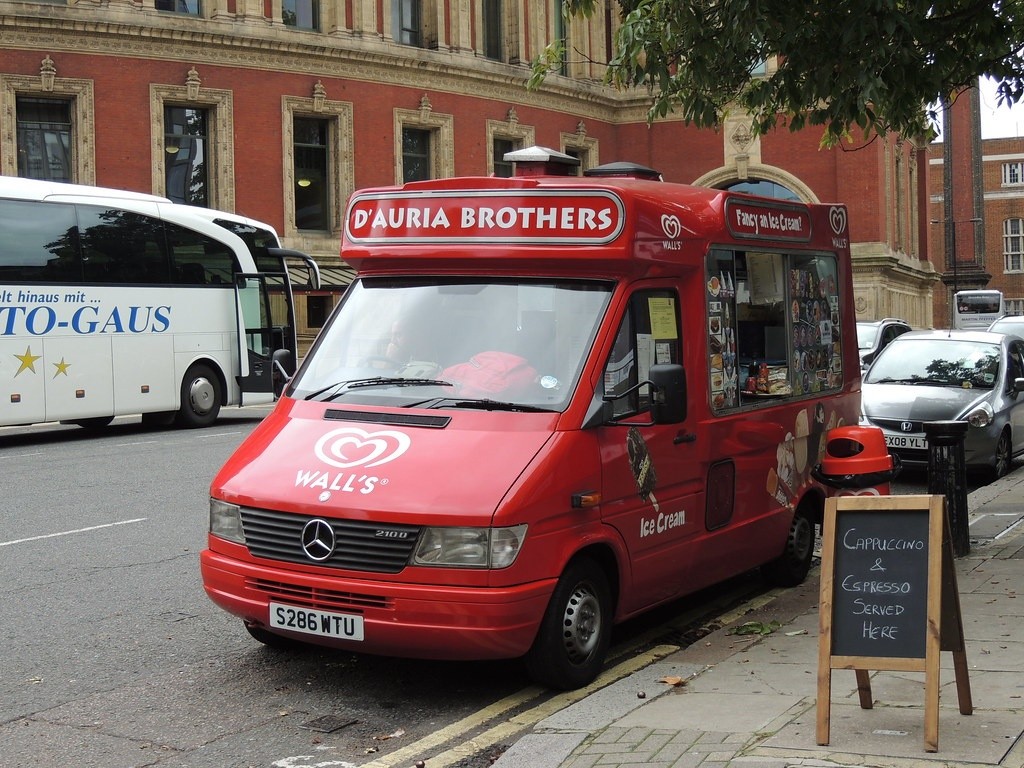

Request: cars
left=857, top=329, right=1024, bottom=477
left=986, top=313, right=1023, bottom=339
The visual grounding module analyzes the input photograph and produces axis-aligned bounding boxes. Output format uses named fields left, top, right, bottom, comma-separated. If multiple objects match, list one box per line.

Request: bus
left=1, top=173, right=323, bottom=436
left=952, top=290, right=1005, bottom=330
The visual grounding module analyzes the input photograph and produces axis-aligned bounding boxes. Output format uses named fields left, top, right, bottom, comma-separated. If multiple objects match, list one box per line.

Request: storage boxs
left=745, top=249, right=784, bottom=307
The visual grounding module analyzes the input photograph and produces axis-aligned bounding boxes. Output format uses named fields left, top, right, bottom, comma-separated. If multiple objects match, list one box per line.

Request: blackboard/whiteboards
left=821, top=496, right=965, bottom=671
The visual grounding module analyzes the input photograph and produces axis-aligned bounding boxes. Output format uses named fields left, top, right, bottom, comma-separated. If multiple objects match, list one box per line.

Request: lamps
left=920, top=419, right=970, bottom=557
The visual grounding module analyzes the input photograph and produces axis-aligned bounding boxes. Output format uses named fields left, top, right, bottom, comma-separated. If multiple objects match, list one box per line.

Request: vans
left=200, top=172, right=864, bottom=692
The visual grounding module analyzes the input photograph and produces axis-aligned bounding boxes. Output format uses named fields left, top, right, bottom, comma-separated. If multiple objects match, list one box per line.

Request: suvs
left=846, top=315, right=914, bottom=379
